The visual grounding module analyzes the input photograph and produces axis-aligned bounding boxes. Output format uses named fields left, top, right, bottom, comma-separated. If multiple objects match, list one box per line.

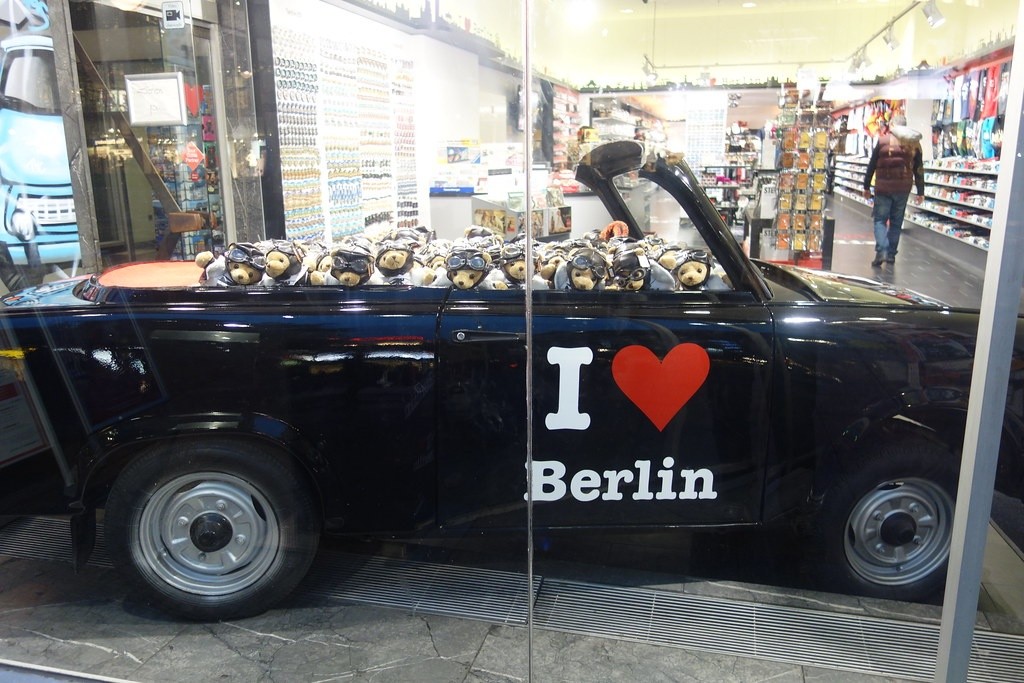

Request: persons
left=632, top=128, right=647, bottom=140
left=863, top=113, right=925, bottom=269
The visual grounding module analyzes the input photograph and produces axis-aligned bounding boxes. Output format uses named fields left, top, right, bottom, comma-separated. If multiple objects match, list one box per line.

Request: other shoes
left=871, top=255, right=884, bottom=266
left=886, top=254, right=897, bottom=264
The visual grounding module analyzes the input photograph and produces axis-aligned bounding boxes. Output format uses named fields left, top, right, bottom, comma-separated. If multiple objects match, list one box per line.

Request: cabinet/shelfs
left=830, top=150, right=1001, bottom=280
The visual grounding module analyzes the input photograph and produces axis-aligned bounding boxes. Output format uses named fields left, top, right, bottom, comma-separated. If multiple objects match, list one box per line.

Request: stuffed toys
left=197, top=223, right=725, bottom=290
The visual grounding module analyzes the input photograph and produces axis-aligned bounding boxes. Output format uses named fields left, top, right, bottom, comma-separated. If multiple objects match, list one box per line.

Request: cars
left=1, top=33, right=81, bottom=274
left=1, top=138, right=1023, bottom=622
